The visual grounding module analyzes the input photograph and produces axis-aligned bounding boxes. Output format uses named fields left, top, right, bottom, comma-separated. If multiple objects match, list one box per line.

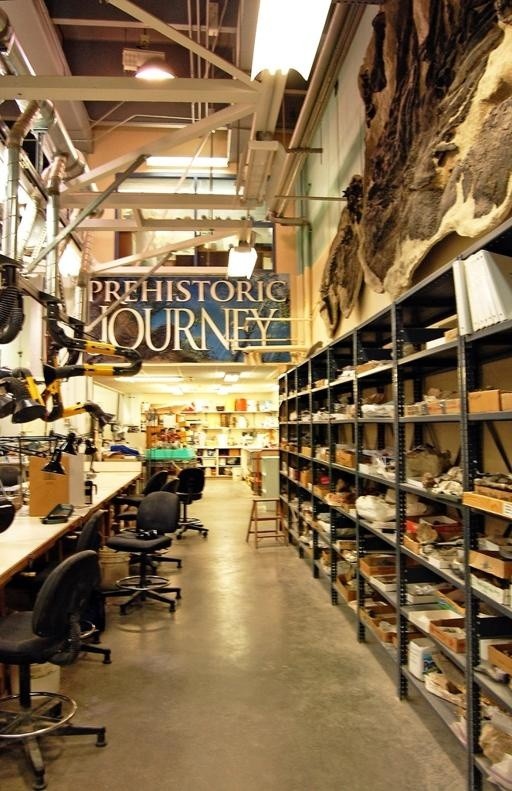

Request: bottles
left=84, top=475, right=97, bottom=504
left=204, top=403, right=208, bottom=411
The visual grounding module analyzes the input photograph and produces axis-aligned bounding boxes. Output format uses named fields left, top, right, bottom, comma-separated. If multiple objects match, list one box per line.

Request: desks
left=0, top=463, right=143, bottom=589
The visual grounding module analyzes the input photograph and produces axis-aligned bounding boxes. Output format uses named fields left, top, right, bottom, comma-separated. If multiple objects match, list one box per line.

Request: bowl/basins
left=216, top=406, right=224, bottom=411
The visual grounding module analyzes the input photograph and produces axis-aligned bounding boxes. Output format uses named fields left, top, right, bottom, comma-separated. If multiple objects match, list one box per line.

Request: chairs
left=0, top=549, right=107, bottom=790
left=102, top=491, right=182, bottom=615
left=126, top=477, right=182, bottom=573
left=173, top=468, right=209, bottom=540
left=111, top=469, right=169, bottom=533
left=14, top=509, right=111, bottom=665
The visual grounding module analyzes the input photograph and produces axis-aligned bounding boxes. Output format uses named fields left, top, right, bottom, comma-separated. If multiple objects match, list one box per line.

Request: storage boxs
left=26, top=453, right=85, bottom=517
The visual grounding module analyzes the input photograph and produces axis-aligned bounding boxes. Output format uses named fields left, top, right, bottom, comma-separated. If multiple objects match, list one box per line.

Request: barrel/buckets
left=99, top=547, right=130, bottom=588
left=235, top=398, right=247, bottom=411
left=10, top=662, right=60, bottom=699
left=220, top=414, right=230, bottom=427
left=232, top=468, right=243, bottom=481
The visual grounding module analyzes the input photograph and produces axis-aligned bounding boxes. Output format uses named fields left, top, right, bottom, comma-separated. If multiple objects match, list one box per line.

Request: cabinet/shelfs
left=275, top=218, right=511, bottom=791
left=142, top=410, right=278, bottom=497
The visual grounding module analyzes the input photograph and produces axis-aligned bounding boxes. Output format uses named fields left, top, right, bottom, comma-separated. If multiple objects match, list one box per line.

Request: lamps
left=128, top=56, right=177, bottom=79
left=226, top=239, right=258, bottom=281
